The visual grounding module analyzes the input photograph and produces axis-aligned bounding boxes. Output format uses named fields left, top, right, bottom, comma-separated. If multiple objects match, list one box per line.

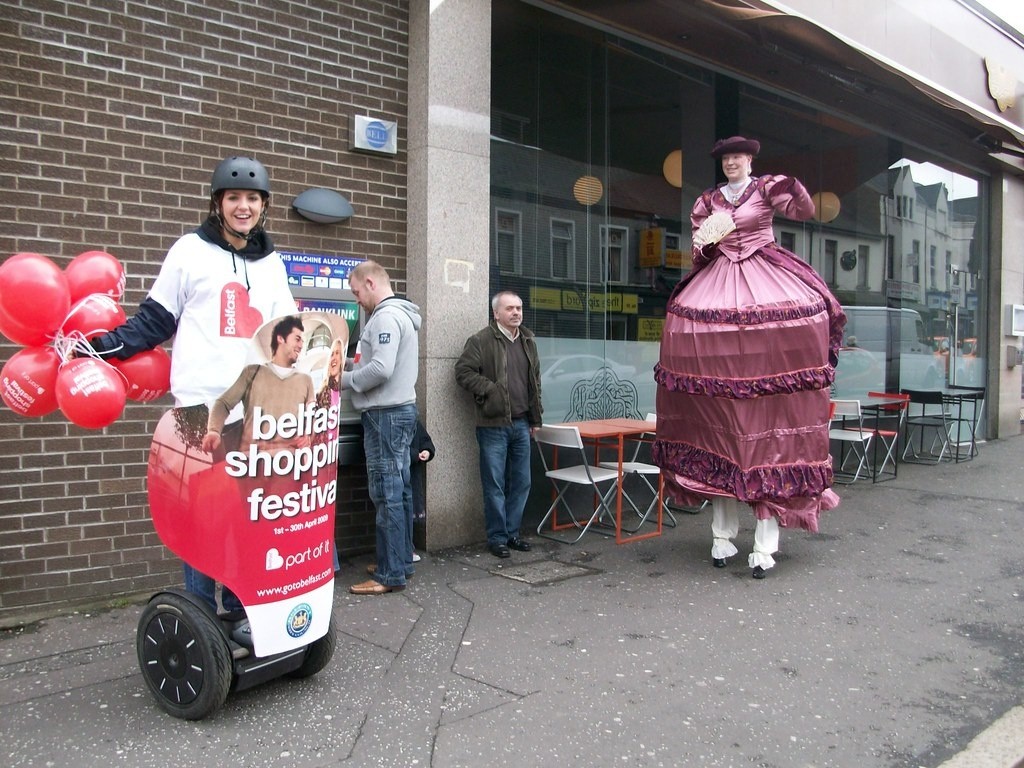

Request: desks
left=551, top=419, right=665, bottom=543
left=914, top=387, right=986, bottom=460
left=829, top=396, right=911, bottom=483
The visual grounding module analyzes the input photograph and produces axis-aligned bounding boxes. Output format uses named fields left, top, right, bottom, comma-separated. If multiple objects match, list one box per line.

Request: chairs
left=825, top=385, right=987, bottom=484
left=599, top=413, right=678, bottom=533
left=530, top=424, right=618, bottom=544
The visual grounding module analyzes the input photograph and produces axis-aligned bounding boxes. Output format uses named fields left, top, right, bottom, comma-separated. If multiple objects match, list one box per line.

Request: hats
left=710, top=135, right=761, bottom=160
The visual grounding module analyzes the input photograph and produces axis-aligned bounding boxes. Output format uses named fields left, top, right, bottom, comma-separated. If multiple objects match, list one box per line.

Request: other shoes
left=412, top=554, right=420, bottom=562
left=221, top=632, right=249, bottom=660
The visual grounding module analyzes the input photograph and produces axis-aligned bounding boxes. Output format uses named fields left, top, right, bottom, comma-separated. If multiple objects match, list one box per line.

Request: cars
left=541, top=353, right=637, bottom=412
left=927, top=337, right=977, bottom=384
left=829, top=348, right=884, bottom=400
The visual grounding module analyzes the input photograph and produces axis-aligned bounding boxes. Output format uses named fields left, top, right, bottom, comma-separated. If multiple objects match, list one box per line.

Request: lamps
left=293, top=189, right=356, bottom=224
left=664, top=149, right=682, bottom=187
left=811, top=191, right=841, bottom=222
left=574, top=176, right=602, bottom=205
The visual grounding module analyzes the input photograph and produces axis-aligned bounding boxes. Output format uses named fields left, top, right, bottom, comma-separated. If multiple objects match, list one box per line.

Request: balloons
left=64, top=294, right=127, bottom=344
left=0, top=346, right=62, bottom=417
left=108, top=345, right=171, bottom=401
left=0, top=253, right=70, bottom=347
left=66, top=251, right=127, bottom=306
left=57, top=357, right=126, bottom=429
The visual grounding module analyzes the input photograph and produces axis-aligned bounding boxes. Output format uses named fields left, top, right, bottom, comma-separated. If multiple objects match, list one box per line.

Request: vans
left=839, top=305, right=940, bottom=389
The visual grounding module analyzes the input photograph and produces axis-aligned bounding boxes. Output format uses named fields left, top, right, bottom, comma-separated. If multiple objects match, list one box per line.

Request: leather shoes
left=506, top=537, right=531, bottom=551
left=368, top=563, right=414, bottom=579
left=490, top=541, right=510, bottom=557
left=351, top=577, right=406, bottom=594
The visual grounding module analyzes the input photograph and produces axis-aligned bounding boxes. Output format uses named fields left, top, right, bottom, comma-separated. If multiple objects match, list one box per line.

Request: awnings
left=684, top=0, right=1024, bottom=153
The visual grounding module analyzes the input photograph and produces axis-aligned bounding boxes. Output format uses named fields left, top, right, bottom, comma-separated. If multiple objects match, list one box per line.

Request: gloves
left=768, top=177, right=795, bottom=198
left=703, top=241, right=721, bottom=260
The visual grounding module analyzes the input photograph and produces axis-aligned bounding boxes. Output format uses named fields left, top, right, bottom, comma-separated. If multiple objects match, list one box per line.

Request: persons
left=202, top=317, right=345, bottom=493
left=342, top=261, right=422, bottom=594
left=62, top=155, right=299, bottom=613
left=653, top=136, right=848, bottom=532
left=409, top=423, right=435, bottom=561
left=455, top=291, right=544, bottom=558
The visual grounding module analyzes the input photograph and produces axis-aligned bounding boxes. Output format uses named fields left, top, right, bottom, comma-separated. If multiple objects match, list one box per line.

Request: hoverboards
left=136, top=587, right=338, bottom=721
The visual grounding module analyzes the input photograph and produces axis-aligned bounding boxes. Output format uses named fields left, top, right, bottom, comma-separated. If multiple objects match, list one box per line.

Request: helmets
left=210, top=155, right=268, bottom=202
